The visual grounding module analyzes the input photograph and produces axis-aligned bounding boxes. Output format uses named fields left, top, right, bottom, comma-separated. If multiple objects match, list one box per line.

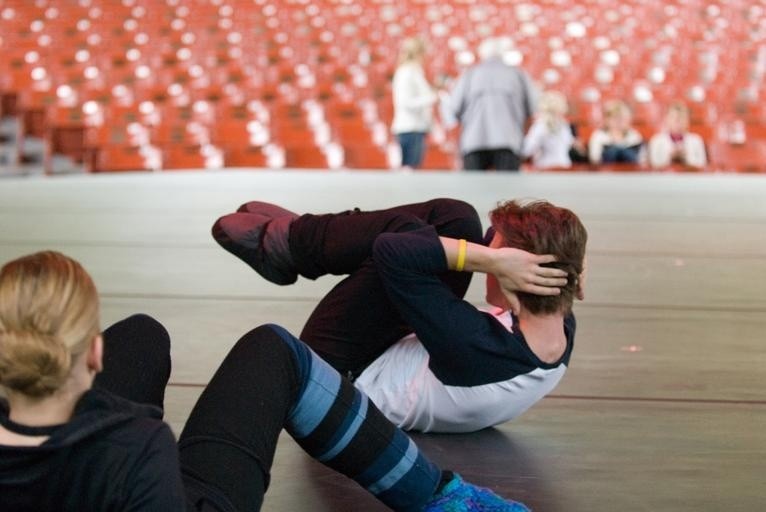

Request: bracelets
left=456, top=237, right=466, bottom=273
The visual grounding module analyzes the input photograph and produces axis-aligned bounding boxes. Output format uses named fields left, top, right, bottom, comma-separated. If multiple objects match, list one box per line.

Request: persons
left=210, top=196, right=589, bottom=434
left=587, top=99, right=647, bottom=168
left=438, top=37, right=542, bottom=171
left=647, top=100, right=707, bottom=169
left=0, top=251, right=532, bottom=512
left=521, top=90, right=586, bottom=170
left=391, top=34, right=443, bottom=168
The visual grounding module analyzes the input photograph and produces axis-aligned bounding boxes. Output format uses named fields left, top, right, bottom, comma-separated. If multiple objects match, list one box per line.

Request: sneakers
left=211, top=200, right=300, bottom=286
left=424, top=471, right=532, bottom=512
